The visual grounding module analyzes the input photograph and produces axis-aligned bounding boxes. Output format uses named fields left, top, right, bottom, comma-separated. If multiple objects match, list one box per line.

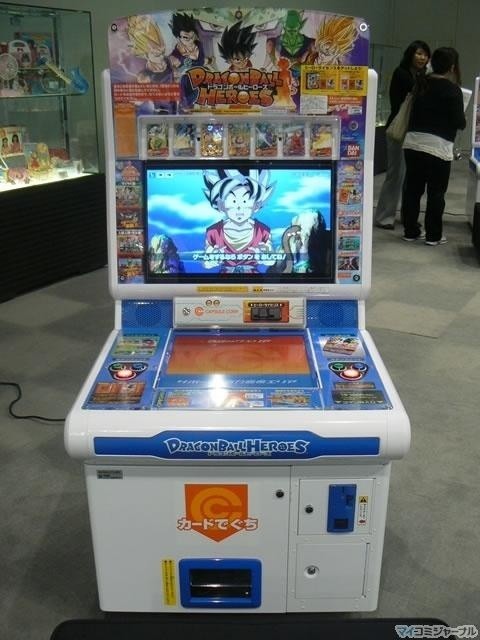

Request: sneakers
left=425, top=236, right=448, bottom=245
left=402, top=232, right=426, bottom=241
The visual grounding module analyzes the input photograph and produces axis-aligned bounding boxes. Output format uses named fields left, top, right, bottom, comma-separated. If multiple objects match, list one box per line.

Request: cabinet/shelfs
left=0, top=3, right=107, bottom=303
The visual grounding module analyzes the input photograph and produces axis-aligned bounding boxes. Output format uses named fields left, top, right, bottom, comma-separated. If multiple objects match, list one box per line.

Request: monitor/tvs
left=143, top=163, right=335, bottom=284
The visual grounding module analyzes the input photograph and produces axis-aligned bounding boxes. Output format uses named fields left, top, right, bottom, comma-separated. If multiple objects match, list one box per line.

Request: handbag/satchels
left=385, top=92, right=414, bottom=143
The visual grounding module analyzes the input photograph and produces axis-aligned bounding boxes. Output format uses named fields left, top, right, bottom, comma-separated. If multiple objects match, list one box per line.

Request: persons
left=373, top=38, right=433, bottom=231
left=400, top=46, right=468, bottom=246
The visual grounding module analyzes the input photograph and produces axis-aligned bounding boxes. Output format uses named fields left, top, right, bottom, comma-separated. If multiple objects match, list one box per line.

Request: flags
left=0, top=30, right=91, bottom=189
